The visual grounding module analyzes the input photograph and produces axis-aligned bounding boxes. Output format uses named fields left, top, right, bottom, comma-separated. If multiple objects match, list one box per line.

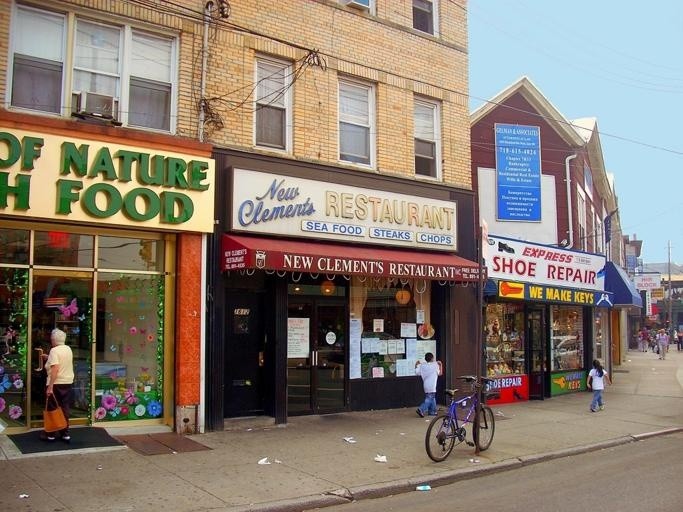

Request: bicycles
left=425, top=375, right=495, bottom=462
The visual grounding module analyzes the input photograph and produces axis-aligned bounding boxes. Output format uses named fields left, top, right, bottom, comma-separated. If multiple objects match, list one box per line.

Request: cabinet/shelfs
left=54, top=296, right=105, bottom=351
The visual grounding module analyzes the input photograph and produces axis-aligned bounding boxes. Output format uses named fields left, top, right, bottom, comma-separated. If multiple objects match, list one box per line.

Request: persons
left=39, top=329, right=75, bottom=441
left=415, top=353, right=443, bottom=418
left=638, top=327, right=683, bottom=360
left=586, top=359, right=612, bottom=412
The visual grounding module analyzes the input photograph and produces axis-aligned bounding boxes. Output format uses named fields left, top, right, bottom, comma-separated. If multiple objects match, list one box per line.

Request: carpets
left=6, top=426, right=126, bottom=456
left=111, top=432, right=214, bottom=456
left=15, top=412, right=79, bottom=424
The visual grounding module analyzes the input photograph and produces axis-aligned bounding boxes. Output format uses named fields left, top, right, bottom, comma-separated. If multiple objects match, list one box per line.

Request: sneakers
left=40, top=435, right=70, bottom=440
left=591, top=405, right=605, bottom=413
left=416, top=408, right=425, bottom=418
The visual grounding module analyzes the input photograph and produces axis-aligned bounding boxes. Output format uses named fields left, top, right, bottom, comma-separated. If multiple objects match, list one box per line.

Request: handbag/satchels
left=43, top=407, right=67, bottom=433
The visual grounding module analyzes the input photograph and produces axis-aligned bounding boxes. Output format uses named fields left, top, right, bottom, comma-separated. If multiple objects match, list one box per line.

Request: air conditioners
left=75, top=90, right=116, bottom=121
